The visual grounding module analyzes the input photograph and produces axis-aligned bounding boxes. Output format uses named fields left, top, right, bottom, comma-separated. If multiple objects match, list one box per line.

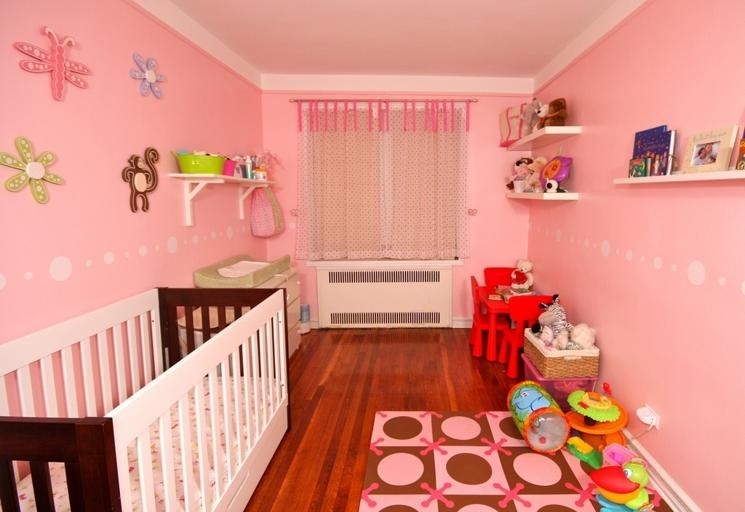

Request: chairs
left=470, top=266, right=559, bottom=380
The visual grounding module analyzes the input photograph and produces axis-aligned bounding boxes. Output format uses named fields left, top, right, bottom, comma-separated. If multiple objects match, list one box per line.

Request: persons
left=692, top=143, right=716, bottom=164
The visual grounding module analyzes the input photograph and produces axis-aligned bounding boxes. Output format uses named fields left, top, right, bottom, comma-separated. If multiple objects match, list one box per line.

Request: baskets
left=522, top=326, right=601, bottom=380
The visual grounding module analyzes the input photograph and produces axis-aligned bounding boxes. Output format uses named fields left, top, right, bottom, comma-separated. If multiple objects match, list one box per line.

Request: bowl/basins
left=172, top=153, right=226, bottom=174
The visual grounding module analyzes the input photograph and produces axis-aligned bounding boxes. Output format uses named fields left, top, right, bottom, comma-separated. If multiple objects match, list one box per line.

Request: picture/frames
left=681, top=124, right=740, bottom=173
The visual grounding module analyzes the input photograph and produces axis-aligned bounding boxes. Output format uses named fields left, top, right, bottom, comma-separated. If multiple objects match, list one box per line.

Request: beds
left=0, top=284, right=289, bottom=512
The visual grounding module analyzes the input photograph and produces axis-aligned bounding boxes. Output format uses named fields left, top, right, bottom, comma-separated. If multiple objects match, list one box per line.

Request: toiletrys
left=245, top=154, right=267, bottom=180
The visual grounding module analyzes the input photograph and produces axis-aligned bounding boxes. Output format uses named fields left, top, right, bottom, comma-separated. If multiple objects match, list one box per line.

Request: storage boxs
left=519, top=351, right=602, bottom=414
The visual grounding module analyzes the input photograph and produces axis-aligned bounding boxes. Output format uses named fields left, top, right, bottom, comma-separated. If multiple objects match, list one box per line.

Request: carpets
left=357, top=409, right=675, bottom=512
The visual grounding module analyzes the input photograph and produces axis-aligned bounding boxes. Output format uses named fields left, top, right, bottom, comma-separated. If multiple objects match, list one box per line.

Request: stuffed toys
left=531, top=294, right=596, bottom=351
left=505, top=155, right=573, bottom=193
left=511, top=259, right=534, bottom=290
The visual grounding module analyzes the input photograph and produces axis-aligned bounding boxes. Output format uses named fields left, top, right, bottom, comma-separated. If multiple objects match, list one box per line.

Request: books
left=627, top=124, right=677, bottom=177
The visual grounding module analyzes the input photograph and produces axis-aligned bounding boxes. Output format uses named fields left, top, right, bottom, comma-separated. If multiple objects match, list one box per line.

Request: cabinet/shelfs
left=507, top=124, right=581, bottom=202
left=257, top=267, right=303, bottom=360
